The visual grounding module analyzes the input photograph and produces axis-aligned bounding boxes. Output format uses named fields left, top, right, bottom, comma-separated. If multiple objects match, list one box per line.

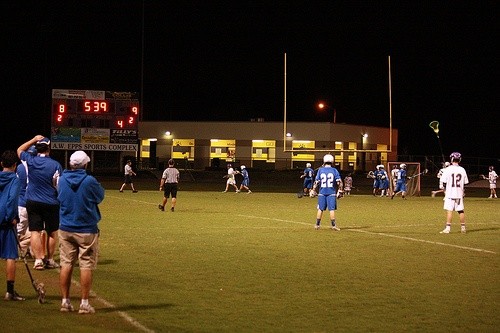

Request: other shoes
left=315, top=225, right=320, bottom=230
left=461, top=226, right=466, bottom=233
left=440, top=228, right=449, bottom=234
left=224, top=191, right=226, bottom=193
left=373, top=192, right=407, bottom=200
left=120, top=190, right=123, bottom=192
left=248, top=191, right=252, bottom=194
left=133, top=190, right=138, bottom=193
left=332, top=226, right=340, bottom=231
left=236, top=190, right=240, bottom=194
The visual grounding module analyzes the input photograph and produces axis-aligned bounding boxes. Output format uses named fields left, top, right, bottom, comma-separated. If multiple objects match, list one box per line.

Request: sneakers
left=4, top=290, right=26, bottom=301
left=79, top=304, right=95, bottom=314
left=59, top=303, right=74, bottom=312
left=46, top=258, right=60, bottom=269
left=33, top=259, right=44, bottom=269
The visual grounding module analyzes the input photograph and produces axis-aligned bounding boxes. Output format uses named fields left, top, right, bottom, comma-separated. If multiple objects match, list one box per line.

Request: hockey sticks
left=429, top=120, right=444, bottom=163
left=431, top=190, right=444, bottom=197
left=11, top=223, right=46, bottom=304
left=408, top=169, right=428, bottom=179
left=480, top=175, right=496, bottom=184
left=300, top=163, right=324, bottom=179
left=297, top=191, right=343, bottom=198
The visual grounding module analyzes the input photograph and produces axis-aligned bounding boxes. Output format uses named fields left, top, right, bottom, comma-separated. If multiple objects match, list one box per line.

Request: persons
left=368, top=164, right=395, bottom=197
left=482, top=166, right=499, bottom=199
left=222, top=164, right=239, bottom=192
left=391, top=164, right=400, bottom=196
left=236, top=165, right=252, bottom=194
left=159, top=159, right=180, bottom=211
left=300, top=162, right=315, bottom=196
left=16, top=135, right=63, bottom=270
left=0, top=151, right=26, bottom=302
left=119, top=160, right=138, bottom=193
left=310, top=154, right=344, bottom=232
left=431, top=161, right=451, bottom=198
left=57, top=150, right=105, bottom=314
left=16, top=145, right=37, bottom=260
left=390, top=164, right=412, bottom=200
left=439, top=152, right=469, bottom=234
left=344, top=171, right=353, bottom=195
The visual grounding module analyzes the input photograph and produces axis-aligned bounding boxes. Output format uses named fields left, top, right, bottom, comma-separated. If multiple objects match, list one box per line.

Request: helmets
left=377, top=164, right=398, bottom=171
left=450, top=152, right=462, bottom=161
left=306, top=163, right=311, bottom=168
left=323, top=154, right=334, bottom=163
left=488, top=166, right=494, bottom=171
left=400, top=163, right=407, bottom=170
left=227, top=164, right=232, bottom=168
left=444, top=162, right=450, bottom=167
left=241, top=165, right=247, bottom=170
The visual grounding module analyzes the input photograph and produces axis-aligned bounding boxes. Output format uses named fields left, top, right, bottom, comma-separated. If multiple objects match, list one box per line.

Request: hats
left=70, top=150, right=91, bottom=165
left=168, top=159, right=175, bottom=165
left=37, top=137, right=51, bottom=144
